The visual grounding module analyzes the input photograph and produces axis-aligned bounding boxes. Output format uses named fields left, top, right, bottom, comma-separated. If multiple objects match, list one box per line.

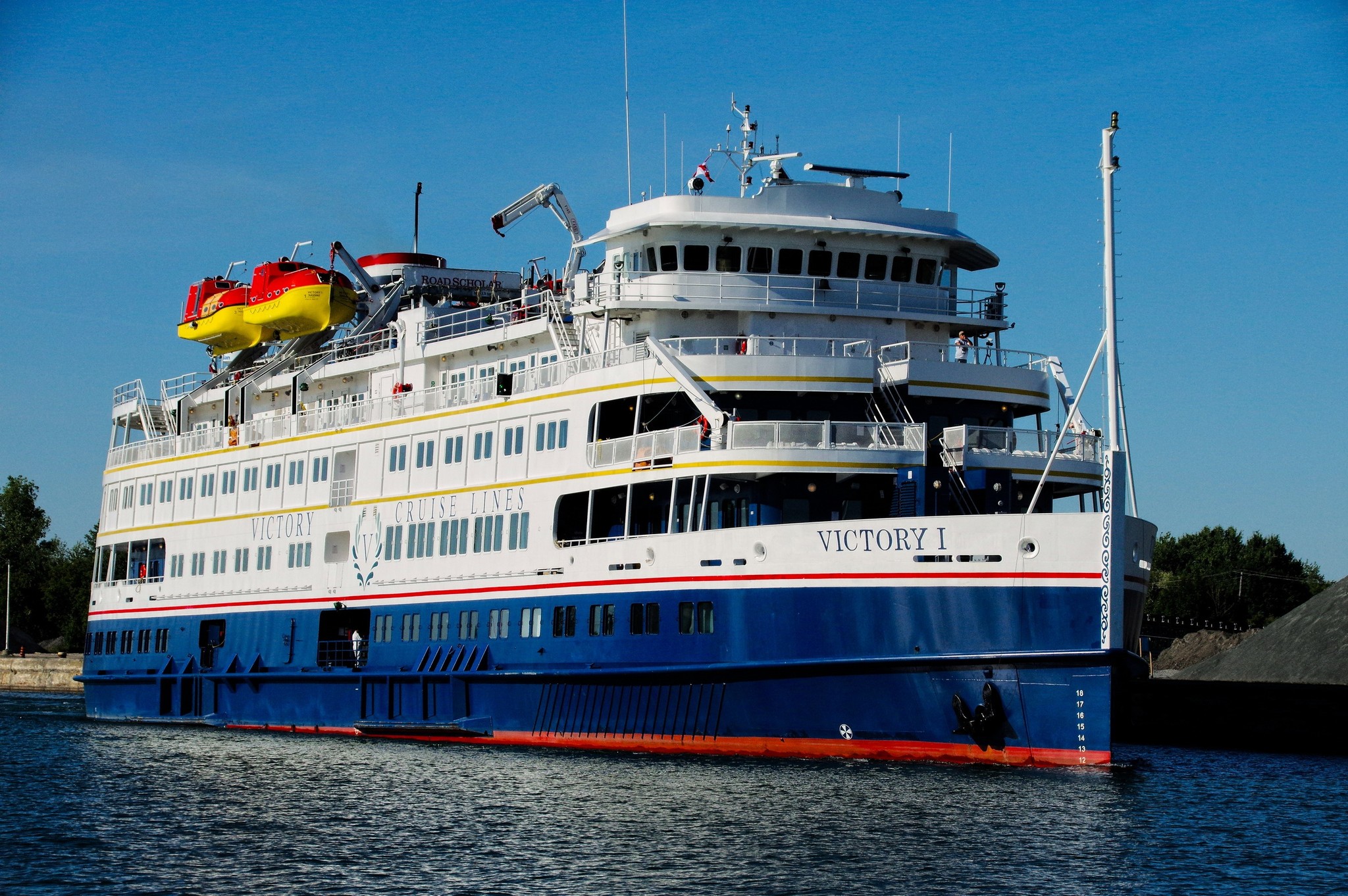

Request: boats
left=71, top=88, right=1162, bottom=773
left=176, top=276, right=276, bottom=356
left=246, top=257, right=357, bottom=339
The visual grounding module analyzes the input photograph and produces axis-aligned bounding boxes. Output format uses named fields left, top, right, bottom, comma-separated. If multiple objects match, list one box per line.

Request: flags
left=693, top=160, right=715, bottom=183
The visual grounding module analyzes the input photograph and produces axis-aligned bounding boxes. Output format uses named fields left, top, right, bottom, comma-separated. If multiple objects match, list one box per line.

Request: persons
left=955, top=331, right=973, bottom=363
left=352, top=627, right=363, bottom=666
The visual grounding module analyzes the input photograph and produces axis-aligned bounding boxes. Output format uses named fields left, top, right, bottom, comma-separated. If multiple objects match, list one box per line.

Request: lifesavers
left=735, top=334, right=747, bottom=355
left=392, top=382, right=403, bottom=400
left=234, top=372, right=241, bottom=380
left=139, top=564, right=147, bottom=579
left=693, top=415, right=708, bottom=440
